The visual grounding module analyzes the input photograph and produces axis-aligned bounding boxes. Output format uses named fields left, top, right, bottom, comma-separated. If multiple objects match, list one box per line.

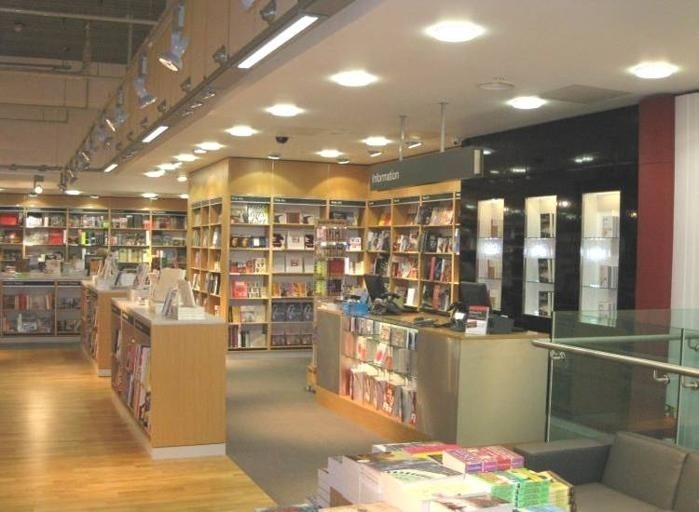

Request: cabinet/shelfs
left=2, top=184, right=233, bottom=462
left=183, top=183, right=643, bottom=465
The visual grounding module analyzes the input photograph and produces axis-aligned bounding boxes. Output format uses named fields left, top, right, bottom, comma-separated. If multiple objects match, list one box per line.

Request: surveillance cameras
left=274, top=135, right=289, bottom=144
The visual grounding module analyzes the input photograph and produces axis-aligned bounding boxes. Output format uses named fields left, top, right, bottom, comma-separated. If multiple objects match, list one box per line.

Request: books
left=228, top=203, right=325, bottom=348
left=68, top=216, right=108, bottom=272
left=1, top=212, right=22, bottom=271
left=26, top=213, right=66, bottom=270
left=191, top=204, right=222, bottom=316
left=152, top=217, right=186, bottom=269
left=315, top=440, right=577, bottom=512
left=2, top=293, right=81, bottom=334
left=111, top=215, right=150, bottom=273
left=329, top=206, right=451, bottom=427
left=464, top=213, right=619, bottom=338
left=81, top=261, right=205, bottom=432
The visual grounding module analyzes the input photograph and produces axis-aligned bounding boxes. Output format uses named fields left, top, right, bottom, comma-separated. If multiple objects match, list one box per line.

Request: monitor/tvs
left=460, top=281, right=493, bottom=315
left=364, top=274, right=387, bottom=303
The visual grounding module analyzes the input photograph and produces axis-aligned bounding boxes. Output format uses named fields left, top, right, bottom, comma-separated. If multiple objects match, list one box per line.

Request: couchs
left=514, top=431, right=699, bottom=511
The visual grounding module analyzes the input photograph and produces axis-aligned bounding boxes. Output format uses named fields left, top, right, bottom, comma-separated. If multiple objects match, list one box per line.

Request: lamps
left=57, top=1, right=189, bottom=192
